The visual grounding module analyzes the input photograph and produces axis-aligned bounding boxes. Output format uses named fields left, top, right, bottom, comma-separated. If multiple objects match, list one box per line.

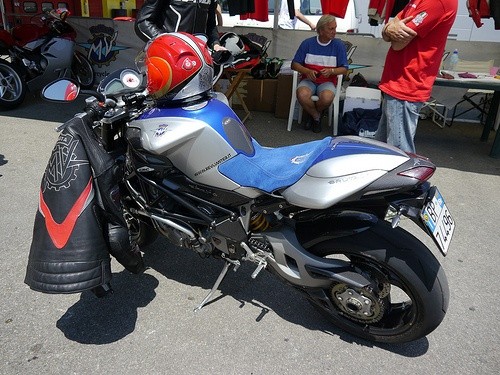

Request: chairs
left=287, top=69, right=343, bottom=136
left=441, top=59, right=495, bottom=128
left=423, top=89, right=448, bottom=130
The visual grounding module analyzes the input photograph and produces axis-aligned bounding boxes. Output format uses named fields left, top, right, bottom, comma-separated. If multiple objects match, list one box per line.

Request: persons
left=372, top=0, right=458, bottom=153
left=135, top=0, right=230, bottom=53
left=291, top=14, right=349, bottom=132
left=278, top=0, right=315, bottom=30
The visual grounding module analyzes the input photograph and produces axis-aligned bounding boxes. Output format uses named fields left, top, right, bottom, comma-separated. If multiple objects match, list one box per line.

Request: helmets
left=135, top=31, right=214, bottom=108
left=54, top=8, right=70, bottom=20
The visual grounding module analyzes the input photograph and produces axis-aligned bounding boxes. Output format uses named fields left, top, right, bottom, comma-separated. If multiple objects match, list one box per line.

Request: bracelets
left=384, top=24, right=389, bottom=38
left=331, top=68, right=336, bottom=75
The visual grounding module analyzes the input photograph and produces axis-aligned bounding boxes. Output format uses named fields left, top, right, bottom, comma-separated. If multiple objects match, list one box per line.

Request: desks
left=213, top=52, right=262, bottom=125
left=432, top=70, right=500, bottom=159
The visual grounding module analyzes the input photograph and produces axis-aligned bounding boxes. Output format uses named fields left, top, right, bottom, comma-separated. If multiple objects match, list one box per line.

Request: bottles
left=448, top=49, right=458, bottom=76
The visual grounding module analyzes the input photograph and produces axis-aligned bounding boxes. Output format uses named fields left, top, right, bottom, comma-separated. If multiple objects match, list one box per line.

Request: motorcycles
left=41, top=0, right=456, bottom=346
left=0, top=8, right=95, bottom=111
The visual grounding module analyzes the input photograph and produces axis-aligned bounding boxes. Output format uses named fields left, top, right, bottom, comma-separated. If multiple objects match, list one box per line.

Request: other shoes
left=311, top=112, right=322, bottom=132
left=304, top=114, right=313, bottom=130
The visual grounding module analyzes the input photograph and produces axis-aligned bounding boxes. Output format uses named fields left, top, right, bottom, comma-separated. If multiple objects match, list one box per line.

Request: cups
left=490, top=68, right=497, bottom=75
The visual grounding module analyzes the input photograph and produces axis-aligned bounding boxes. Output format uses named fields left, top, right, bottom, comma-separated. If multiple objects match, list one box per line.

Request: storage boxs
left=342, top=86, right=382, bottom=134
left=231, top=76, right=293, bottom=118
